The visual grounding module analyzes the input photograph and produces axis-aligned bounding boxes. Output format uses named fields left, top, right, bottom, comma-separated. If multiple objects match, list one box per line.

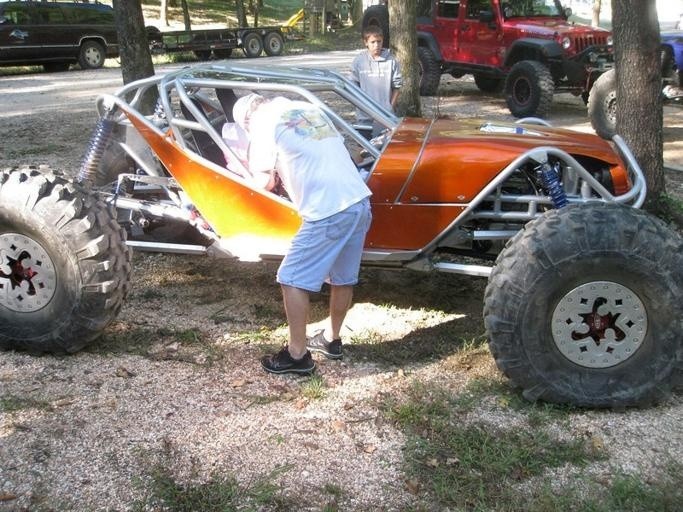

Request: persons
left=346, top=24, right=404, bottom=164
left=229, top=86, right=375, bottom=379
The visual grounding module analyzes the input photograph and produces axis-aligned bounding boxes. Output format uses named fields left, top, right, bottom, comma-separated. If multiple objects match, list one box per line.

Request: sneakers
left=305, top=328, right=343, bottom=361
left=258, top=343, right=316, bottom=374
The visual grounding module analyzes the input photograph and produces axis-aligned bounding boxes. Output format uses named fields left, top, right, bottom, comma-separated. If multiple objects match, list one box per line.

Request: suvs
left=0, top=63, right=683, bottom=409
left=0, top=0, right=118, bottom=73
left=658, top=12, right=682, bottom=106
left=363, top=1, right=615, bottom=119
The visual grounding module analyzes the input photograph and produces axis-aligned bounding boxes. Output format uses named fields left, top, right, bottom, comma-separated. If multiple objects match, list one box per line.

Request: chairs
left=181, top=93, right=227, bottom=168
left=216, top=89, right=260, bottom=122
